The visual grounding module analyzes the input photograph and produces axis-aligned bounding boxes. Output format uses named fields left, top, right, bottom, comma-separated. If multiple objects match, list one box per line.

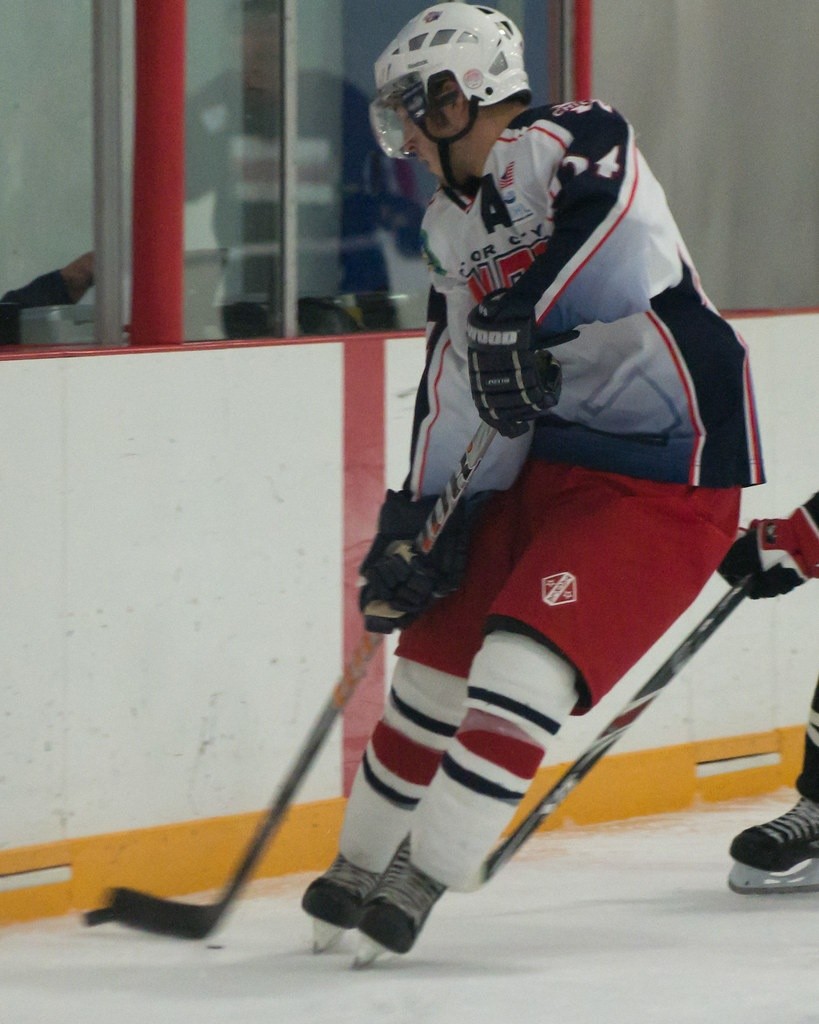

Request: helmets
left=372, top=0, right=534, bottom=110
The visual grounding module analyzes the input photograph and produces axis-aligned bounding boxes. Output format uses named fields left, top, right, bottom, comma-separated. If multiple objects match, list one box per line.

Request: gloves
left=357, top=490, right=465, bottom=635
left=714, top=503, right=818, bottom=599
left=462, top=291, right=563, bottom=444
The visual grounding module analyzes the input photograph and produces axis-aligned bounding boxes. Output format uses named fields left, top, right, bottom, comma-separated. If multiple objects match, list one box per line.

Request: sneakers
left=304, top=852, right=381, bottom=960
left=729, top=798, right=819, bottom=895
left=354, top=829, right=444, bottom=972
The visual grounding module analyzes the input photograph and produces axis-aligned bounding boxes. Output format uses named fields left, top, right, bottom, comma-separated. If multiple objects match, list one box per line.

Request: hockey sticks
left=106, top=420, right=501, bottom=941
left=476, top=570, right=753, bottom=883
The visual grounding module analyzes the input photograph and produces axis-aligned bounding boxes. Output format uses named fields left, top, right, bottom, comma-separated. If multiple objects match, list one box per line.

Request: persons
left=715, top=490, right=819, bottom=889
left=1, top=245, right=95, bottom=343
left=182, top=0, right=428, bottom=340
left=299, top=6, right=765, bottom=959
